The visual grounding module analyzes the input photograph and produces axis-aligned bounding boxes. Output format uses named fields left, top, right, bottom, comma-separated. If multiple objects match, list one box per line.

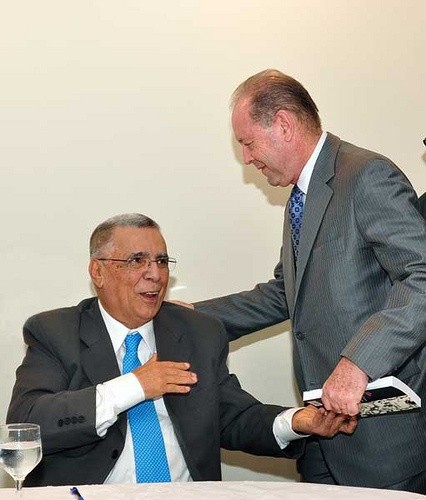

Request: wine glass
left=0, top=423, right=42, bottom=500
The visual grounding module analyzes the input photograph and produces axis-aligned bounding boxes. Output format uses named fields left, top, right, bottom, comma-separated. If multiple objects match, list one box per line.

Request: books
left=303, top=375, right=421, bottom=418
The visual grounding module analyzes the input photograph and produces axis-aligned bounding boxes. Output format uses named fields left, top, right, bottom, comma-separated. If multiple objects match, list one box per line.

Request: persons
left=5, top=212, right=358, bottom=487
left=161, top=69, right=426, bottom=495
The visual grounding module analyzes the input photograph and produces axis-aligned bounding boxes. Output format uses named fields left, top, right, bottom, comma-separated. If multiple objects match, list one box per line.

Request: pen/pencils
left=71, top=486, right=84, bottom=500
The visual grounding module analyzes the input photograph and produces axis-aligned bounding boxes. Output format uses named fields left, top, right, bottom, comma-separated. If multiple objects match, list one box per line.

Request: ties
left=287, top=185, right=304, bottom=268
left=122, top=333, right=172, bottom=483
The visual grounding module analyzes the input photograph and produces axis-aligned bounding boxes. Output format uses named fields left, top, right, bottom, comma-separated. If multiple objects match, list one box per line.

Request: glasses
left=100, top=256, right=176, bottom=273
